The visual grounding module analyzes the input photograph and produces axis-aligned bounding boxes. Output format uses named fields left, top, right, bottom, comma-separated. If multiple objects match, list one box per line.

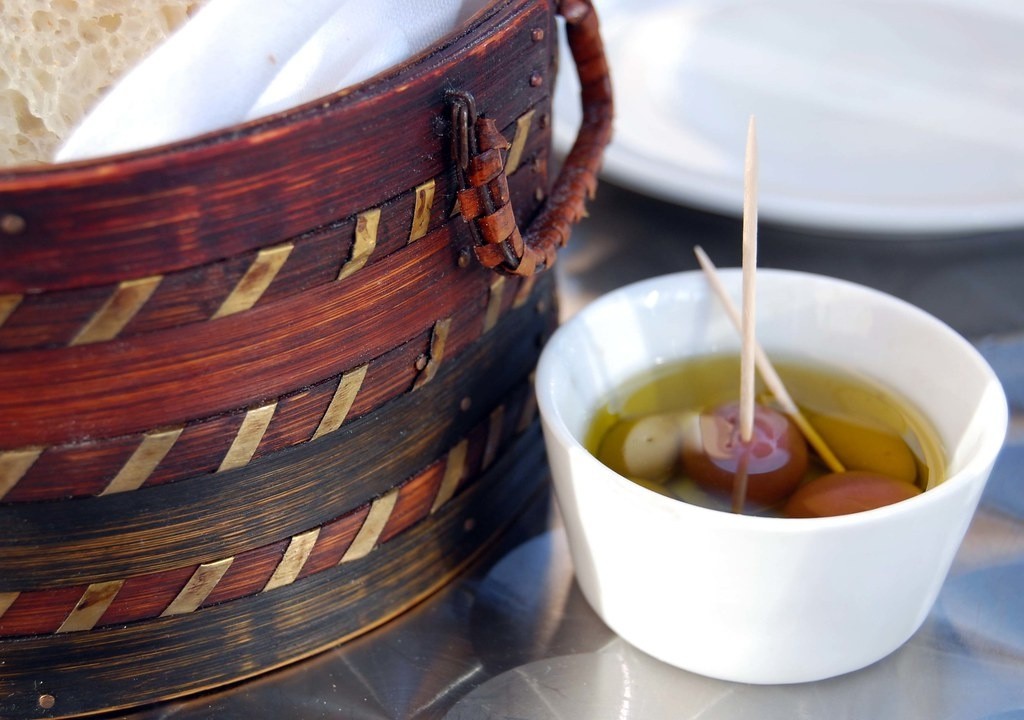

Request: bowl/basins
left=534, top=266, right=1008, bottom=684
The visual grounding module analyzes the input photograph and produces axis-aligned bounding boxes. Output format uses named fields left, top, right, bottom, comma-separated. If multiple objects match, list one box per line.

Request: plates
left=552, top=0, right=1024, bottom=232
